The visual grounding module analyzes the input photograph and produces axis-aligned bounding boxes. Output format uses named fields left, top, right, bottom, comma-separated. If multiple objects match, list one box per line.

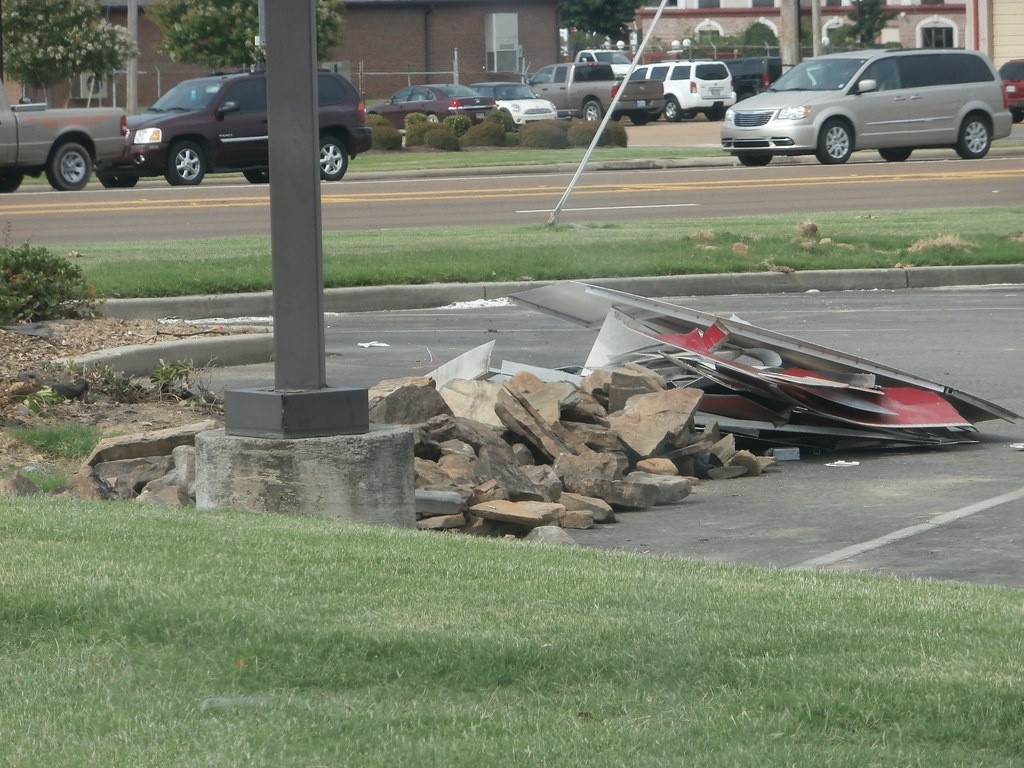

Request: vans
left=715, top=55, right=783, bottom=103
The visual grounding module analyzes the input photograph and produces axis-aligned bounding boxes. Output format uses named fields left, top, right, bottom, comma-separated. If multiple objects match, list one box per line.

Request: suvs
left=720, top=46, right=1013, bottom=167
left=618, top=58, right=737, bottom=123
left=96, top=68, right=373, bottom=188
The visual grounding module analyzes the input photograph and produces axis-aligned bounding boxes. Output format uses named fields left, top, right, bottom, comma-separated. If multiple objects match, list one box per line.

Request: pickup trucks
left=521, top=61, right=667, bottom=126
left=0, top=77, right=128, bottom=194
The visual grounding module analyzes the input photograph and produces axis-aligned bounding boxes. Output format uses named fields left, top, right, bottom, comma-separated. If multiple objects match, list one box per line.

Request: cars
left=364, top=83, right=497, bottom=127
left=997, top=58, right=1024, bottom=124
left=466, top=81, right=558, bottom=127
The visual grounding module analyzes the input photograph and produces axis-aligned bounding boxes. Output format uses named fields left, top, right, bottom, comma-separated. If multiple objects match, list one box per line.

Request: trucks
left=570, top=49, right=632, bottom=81
